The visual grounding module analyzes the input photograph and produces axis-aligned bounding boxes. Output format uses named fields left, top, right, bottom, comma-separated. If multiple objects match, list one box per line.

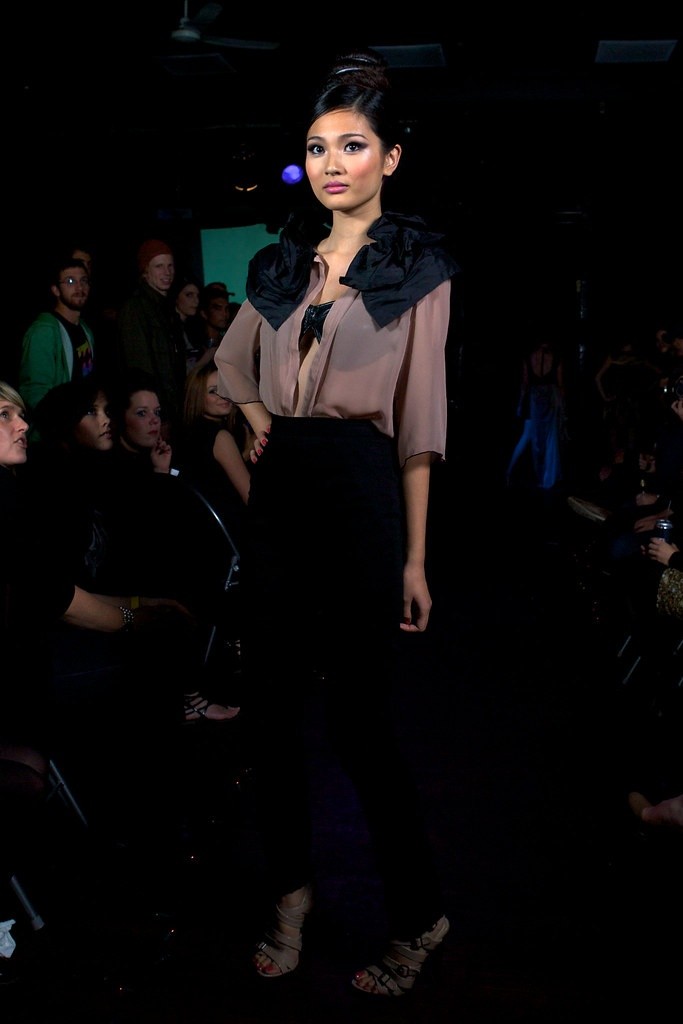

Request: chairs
left=191, top=485, right=242, bottom=666
left=0, top=742, right=88, bottom=930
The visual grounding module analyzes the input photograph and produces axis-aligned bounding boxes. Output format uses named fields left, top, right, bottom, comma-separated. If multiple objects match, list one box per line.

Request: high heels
left=350, top=915, right=451, bottom=995
left=251, top=883, right=312, bottom=977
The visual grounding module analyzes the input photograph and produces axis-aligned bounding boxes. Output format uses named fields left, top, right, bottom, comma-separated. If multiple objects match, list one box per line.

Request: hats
left=138, top=239, right=173, bottom=265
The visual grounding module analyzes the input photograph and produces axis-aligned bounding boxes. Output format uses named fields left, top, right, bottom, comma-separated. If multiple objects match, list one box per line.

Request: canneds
left=653, top=519, right=673, bottom=546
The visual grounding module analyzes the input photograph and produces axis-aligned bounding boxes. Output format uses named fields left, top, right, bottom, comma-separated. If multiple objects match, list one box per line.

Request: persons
left=508, top=329, right=683, bottom=829
left=213, top=54, right=462, bottom=997
left=19, top=237, right=258, bottom=677
left=0, top=384, right=242, bottom=987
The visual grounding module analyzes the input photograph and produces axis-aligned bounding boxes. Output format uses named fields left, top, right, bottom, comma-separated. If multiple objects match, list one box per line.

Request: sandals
left=184, top=692, right=241, bottom=723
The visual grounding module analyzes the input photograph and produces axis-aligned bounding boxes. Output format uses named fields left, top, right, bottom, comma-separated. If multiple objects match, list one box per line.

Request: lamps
left=166, top=0, right=201, bottom=42
left=230, top=144, right=259, bottom=193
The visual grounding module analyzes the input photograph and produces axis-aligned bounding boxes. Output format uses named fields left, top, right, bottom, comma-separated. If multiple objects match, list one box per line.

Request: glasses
left=58, top=277, right=88, bottom=286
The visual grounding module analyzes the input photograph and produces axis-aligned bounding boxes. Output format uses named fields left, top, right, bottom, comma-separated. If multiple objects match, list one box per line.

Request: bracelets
left=131, top=597, right=138, bottom=609
left=119, top=606, right=135, bottom=630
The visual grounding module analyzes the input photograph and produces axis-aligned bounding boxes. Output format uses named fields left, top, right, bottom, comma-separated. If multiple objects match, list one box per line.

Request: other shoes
left=565, top=496, right=608, bottom=522
